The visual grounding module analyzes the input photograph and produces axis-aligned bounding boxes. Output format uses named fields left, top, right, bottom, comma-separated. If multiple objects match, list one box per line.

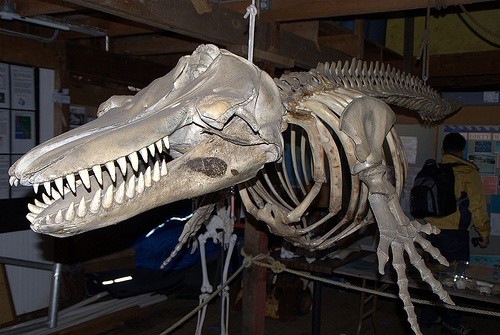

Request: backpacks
left=410, top=158, right=468, bottom=219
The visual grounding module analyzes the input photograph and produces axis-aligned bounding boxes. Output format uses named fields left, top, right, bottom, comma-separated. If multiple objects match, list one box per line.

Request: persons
left=424, top=132, right=491, bottom=334
left=83, top=122, right=343, bottom=310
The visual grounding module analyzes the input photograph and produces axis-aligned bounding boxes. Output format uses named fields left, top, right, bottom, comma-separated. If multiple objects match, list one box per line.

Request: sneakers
left=442, top=319, right=473, bottom=335
left=422, top=317, right=441, bottom=324
